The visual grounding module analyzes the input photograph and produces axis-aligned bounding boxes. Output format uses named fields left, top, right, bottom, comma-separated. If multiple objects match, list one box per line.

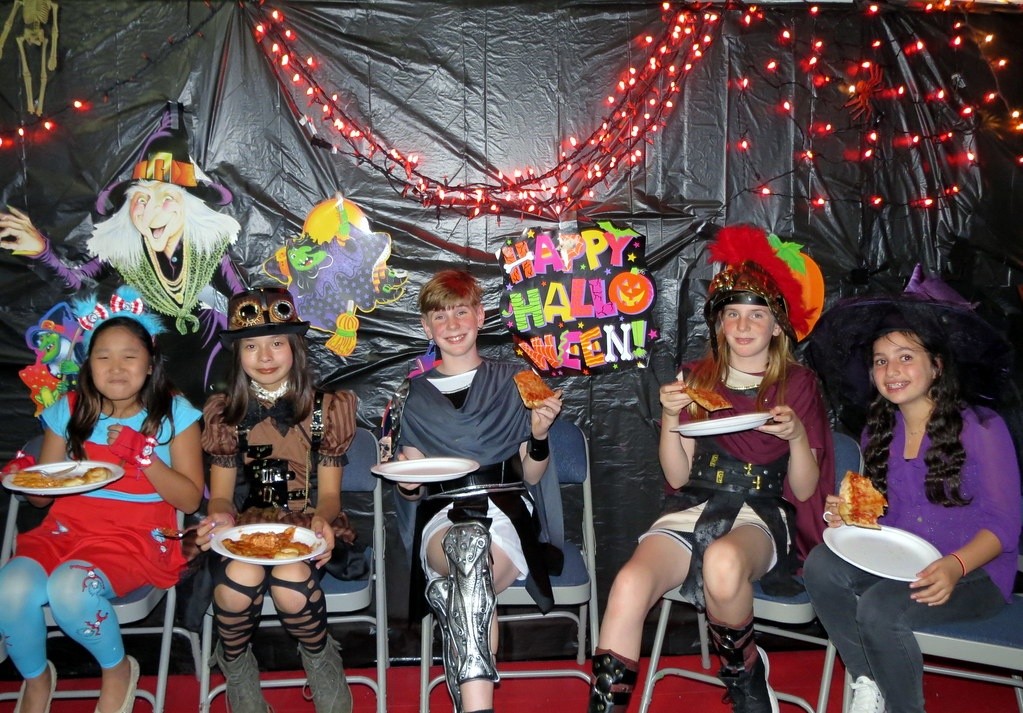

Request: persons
left=587, top=261, right=840, bottom=713
left=802, top=262, right=1023, bottom=713
left=0, top=286, right=204, bottom=713
left=394, top=268, right=564, bottom=713
left=194, top=285, right=356, bottom=713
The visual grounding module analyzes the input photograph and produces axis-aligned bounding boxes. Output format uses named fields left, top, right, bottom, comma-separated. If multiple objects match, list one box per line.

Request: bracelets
left=226, top=512, right=236, bottom=525
left=528, top=433, right=550, bottom=461
left=950, top=552, right=967, bottom=577
left=398, top=484, right=422, bottom=496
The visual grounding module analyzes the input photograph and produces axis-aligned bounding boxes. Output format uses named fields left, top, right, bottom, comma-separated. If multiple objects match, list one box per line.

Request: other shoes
left=14, top=660, right=57, bottom=713
left=93, top=654, right=140, bottom=713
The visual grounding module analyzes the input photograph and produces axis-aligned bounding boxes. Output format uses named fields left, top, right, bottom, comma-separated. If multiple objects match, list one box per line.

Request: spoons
left=155, top=520, right=225, bottom=539
left=0, top=466, right=77, bottom=477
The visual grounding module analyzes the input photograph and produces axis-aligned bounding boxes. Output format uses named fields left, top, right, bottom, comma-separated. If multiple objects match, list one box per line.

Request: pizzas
left=13, top=470, right=61, bottom=488
left=684, top=386, right=733, bottom=411
left=222, top=525, right=297, bottom=559
left=838, top=470, right=888, bottom=529
left=514, top=370, right=556, bottom=408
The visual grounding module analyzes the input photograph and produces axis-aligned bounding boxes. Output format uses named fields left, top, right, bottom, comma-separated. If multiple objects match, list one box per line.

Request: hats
left=812, top=263, right=1012, bottom=410
left=704, top=226, right=816, bottom=349
left=219, top=287, right=310, bottom=351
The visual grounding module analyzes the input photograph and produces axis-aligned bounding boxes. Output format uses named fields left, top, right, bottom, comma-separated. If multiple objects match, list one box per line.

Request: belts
left=689, top=452, right=785, bottom=495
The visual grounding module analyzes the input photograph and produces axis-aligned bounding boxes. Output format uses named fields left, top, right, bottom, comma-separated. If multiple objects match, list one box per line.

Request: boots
left=208, top=638, right=269, bottom=713
left=298, top=633, right=353, bottom=713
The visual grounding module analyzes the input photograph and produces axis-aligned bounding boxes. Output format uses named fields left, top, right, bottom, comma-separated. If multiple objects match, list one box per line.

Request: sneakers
left=848, top=676, right=885, bottom=713
left=718, top=645, right=780, bottom=713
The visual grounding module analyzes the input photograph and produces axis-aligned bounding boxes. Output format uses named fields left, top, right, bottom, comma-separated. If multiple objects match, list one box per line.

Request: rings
left=822, top=512, right=833, bottom=522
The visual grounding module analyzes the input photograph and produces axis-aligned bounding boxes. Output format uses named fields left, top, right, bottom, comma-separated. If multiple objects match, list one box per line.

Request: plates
left=2, top=461, right=126, bottom=495
left=822, top=524, right=944, bottom=582
left=210, top=523, right=327, bottom=566
left=669, top=413, right=773, bottom=437
left=371, top=457, right=479, bottom=482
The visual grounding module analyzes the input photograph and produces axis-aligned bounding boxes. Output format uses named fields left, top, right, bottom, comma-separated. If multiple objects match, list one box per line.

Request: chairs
left=195, top=421, right=378, bottom=713
left=839, top=548, right=1023, bottom=713
left=392, top=411, right=594, bottom=713
left=639, top=433, right=865, bottom=713
left=1, top=434, right=197, bottom=713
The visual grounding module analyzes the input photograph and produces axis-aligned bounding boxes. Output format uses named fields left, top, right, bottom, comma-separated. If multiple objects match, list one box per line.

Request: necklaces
left=905, top=425, right=926, bottom=436
